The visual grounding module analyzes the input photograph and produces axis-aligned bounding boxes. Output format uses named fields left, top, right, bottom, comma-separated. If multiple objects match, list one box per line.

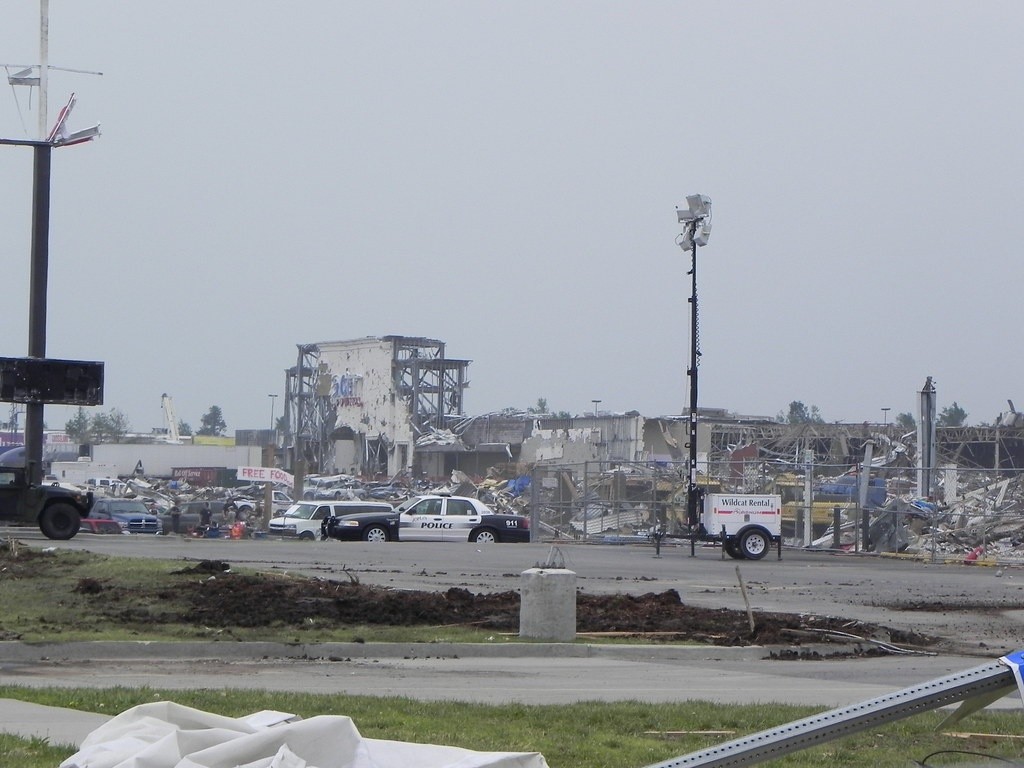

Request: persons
left=170, top=501, right=182, bottom=533
left=248, top=500, right=262, bottom=518
left=199, top=502, right=213, bottom=526
left=486, top=458, right=511, bottom=480
left=223, top=498, right=239, bottom=518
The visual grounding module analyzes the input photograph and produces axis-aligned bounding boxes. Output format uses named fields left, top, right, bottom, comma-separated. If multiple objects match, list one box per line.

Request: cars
left=80, top=519, right=124, bottom=535
left=319, top=494, right=531, bottom=545
left=303, top=472, right=415, bottom=502
left=159, top=499, right=230, bottom=535
left=86, top=497, right=164, bottom=535
left=89, top=477, right=131, bottom=493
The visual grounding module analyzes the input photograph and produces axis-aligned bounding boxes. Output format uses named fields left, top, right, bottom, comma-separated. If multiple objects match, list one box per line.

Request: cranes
left=160, top=392, right=184, bottom=445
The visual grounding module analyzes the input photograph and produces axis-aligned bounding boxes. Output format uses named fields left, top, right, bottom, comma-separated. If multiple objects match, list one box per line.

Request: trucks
left=232, top=489, right=295, bottom=521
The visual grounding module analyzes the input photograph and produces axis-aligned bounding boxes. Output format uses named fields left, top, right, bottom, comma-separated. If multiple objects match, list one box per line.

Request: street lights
left=675, top=193, right=713, bottom=535
left=268, top=394, right=278, bottom=469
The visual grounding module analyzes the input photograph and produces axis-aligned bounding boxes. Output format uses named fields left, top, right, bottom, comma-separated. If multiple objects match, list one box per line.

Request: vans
left=267, top=499, right=395, bottom=542
